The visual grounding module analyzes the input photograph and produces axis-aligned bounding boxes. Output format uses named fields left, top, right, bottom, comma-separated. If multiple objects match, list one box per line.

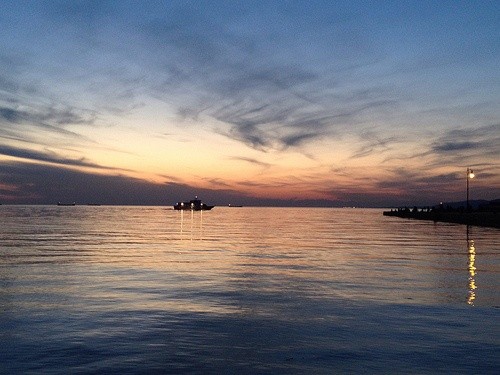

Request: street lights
left=465, top=168, right=475, bottom=211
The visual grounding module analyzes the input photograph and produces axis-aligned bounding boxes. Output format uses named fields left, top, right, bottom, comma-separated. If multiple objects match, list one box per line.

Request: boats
left=172, top=196, right=215, bottom=211
left=88, top=203, right=101, bottom=206
left=229, top=205, right=244, bottom=207
left=57, top=201, right=75, bottom=206
